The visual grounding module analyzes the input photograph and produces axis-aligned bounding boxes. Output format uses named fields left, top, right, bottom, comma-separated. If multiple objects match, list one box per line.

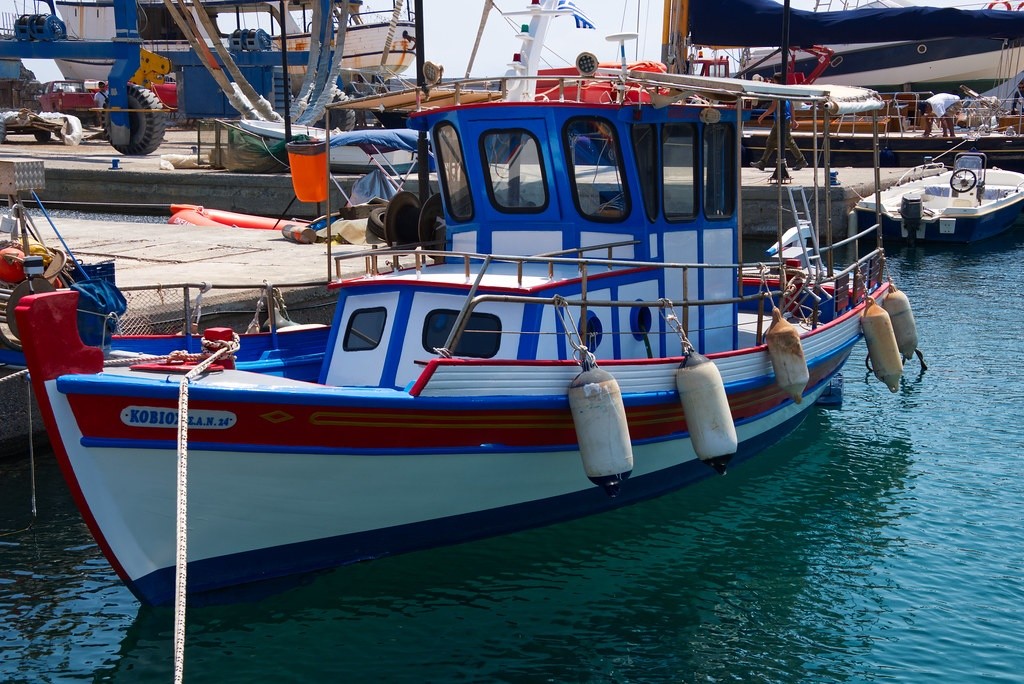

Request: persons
left=93, top=81, right=109, bottom=110
left=750, top=74, right=809, bottom=172
left=920, top=93, right=962, bottom=137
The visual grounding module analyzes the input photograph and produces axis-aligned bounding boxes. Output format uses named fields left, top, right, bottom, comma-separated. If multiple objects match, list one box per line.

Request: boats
left=854, top=145, right=1024, bottom=247
left=9, top=0, right=928, bottom=610
left=273, top=0, right=416, bottom=83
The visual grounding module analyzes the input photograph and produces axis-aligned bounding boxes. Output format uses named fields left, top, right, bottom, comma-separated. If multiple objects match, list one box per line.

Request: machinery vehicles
left=0, top=0, right=357, bottom=157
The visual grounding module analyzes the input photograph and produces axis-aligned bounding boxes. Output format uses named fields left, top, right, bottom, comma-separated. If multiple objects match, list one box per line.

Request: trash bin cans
left=284, top=141, right=327, bottom=202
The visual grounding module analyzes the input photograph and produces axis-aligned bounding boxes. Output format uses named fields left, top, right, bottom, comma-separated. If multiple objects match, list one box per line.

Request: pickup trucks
left=35, top=79, right=99, bottom=126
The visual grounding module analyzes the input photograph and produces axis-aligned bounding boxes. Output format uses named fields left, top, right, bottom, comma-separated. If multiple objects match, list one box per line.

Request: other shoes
left=750, top=160, right=765, bottom=172
left=792, top=158, right=809, bottom=171
left=919, top=133, right=930, bottom=138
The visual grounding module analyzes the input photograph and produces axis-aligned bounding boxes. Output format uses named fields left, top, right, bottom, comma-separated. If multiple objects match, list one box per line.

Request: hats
left=919, top=100, right=927, bottom=116
